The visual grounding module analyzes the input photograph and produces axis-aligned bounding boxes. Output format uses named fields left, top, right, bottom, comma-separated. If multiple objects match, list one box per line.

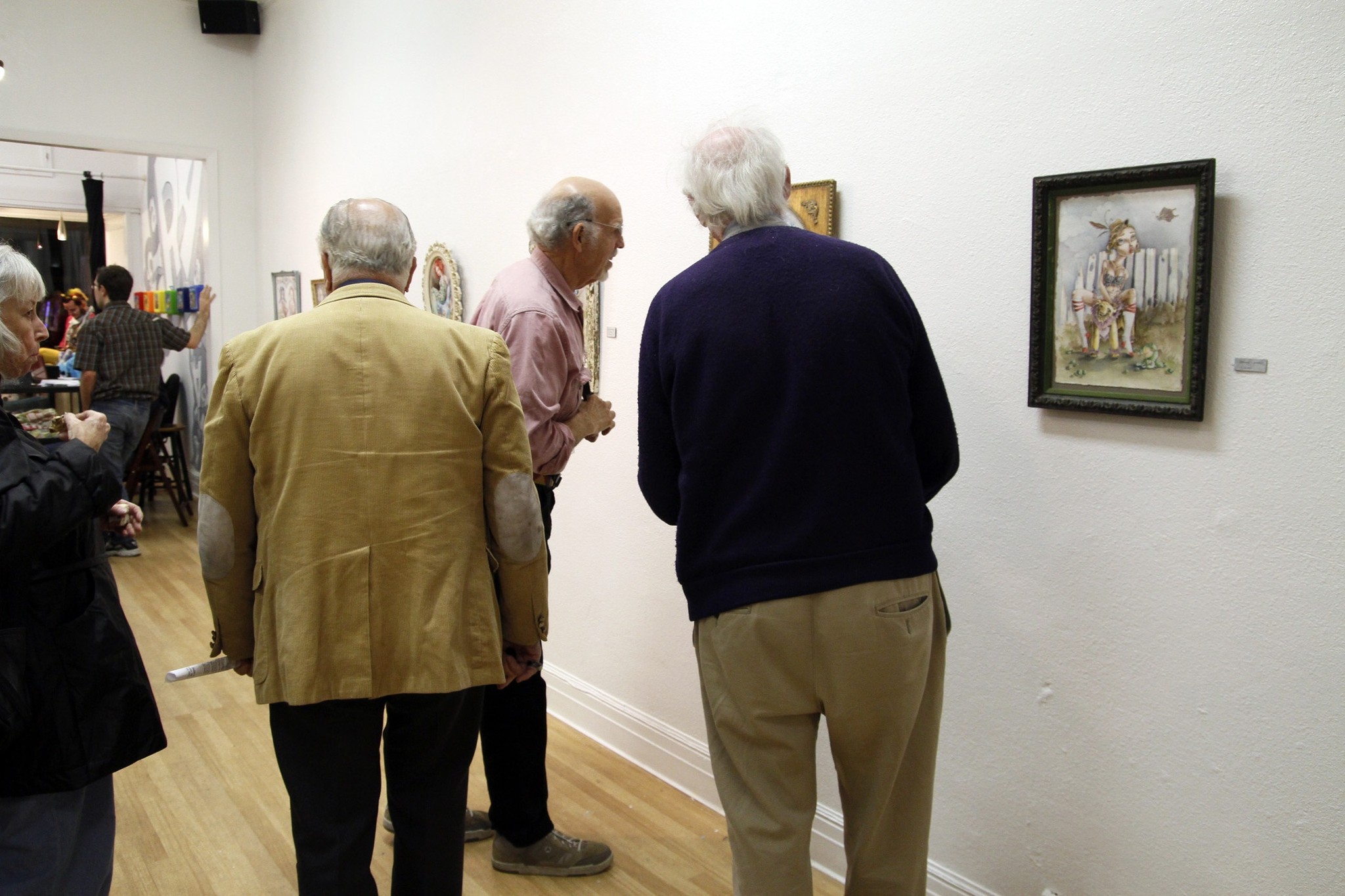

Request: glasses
left=567, top=218, right=625, bottom=238
left=91, top=283, right=103, bottom=289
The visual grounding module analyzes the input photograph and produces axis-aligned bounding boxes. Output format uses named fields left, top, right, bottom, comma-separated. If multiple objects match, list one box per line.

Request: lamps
left=57, top=211, right=67, bottom=240
left=37, top=220, right=43, bottom=249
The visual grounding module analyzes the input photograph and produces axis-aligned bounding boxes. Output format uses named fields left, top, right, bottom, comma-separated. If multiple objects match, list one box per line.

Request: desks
left=0, top=377, right=83, bottom=414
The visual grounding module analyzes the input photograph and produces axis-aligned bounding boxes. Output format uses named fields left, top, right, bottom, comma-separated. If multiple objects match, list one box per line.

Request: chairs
left=123, top=373, right=194, bottom=526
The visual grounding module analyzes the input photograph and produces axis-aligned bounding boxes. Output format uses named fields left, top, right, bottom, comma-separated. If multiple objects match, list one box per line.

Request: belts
left=533, top=471, right=562, bottom=488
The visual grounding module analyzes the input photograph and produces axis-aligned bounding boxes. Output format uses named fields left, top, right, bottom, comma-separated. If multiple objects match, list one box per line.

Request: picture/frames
left=270, top=270, right=300, bottom=321
left=310, top=278, right=327, bottom=308
left=573, top=281, right=600, bottom=396
left=708, top=179, right=837, bottom=253
left=1028, top=158, right=1216, bottom=422
left=422, top=240, right=463, bottom=323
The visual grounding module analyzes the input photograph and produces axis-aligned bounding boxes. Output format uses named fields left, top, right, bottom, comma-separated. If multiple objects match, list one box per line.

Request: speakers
left=197, top=0, right=260, bottom=34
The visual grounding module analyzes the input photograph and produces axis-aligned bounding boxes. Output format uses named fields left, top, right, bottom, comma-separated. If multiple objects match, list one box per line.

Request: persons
left=0, top=246, right=168, bottom=896
left=636, top=123, right=960, bottom=896
left=59, top=287, right=96, bottom=353
left=384, top=178, right=613, bottom=875
left=71, top=265, right=217, bottom=557
left=198, top=198, right=548, bottom=896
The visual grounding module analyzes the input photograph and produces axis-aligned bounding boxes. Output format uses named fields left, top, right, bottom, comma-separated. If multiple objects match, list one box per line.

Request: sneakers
left=383, top=803, right=495, bottom=841
left=492, top=825, right=614, bottom=876
left=104, top=536, right=140, bottom=557
